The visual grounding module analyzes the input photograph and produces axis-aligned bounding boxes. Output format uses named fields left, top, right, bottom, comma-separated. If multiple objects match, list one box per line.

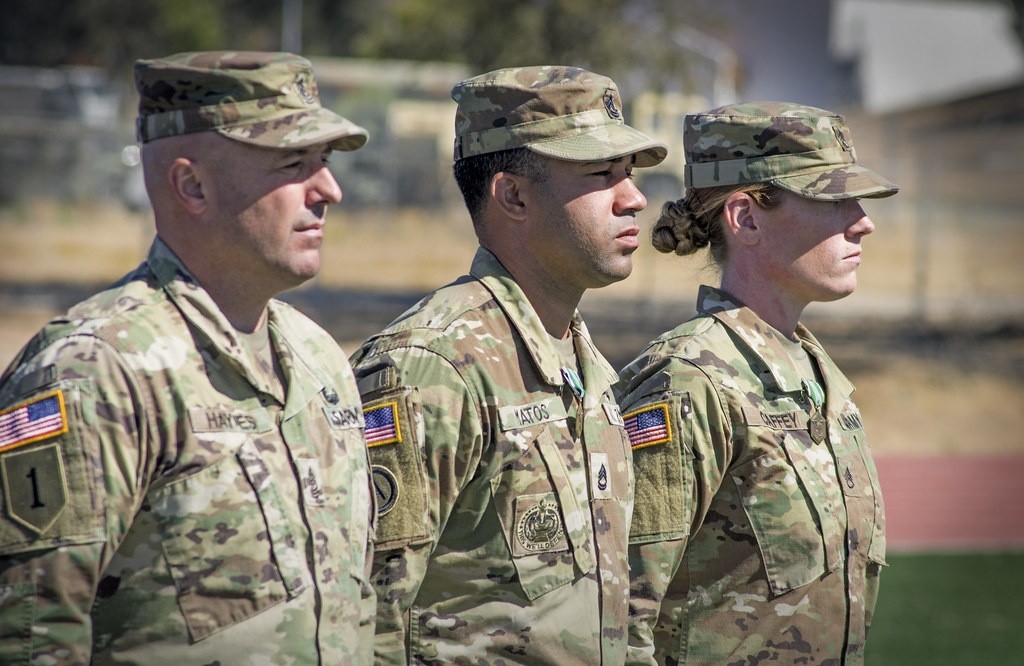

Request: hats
left=683, top=101, right=900, bottom=200
left=134, top=51, right=369, bottom=152
left=451, top=65, right=667, bottom=169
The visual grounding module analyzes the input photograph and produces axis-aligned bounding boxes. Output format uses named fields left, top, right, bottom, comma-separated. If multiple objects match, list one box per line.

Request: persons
left=346, top=65, right=670, bottom=666
left=611, top=100, right=900, bottom=666
left=0, top=50, right=378, bottom=666
